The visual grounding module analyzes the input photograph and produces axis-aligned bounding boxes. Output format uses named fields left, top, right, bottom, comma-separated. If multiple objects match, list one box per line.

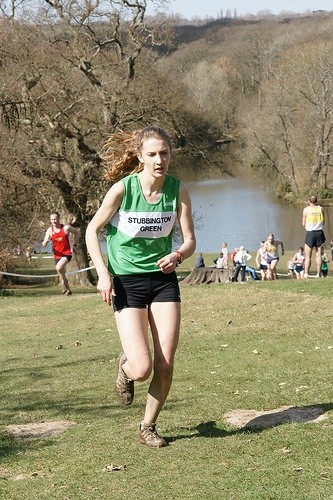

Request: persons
left=197, top=233, right=333, bottom=282
left=26, top=247, right=33, bottom=264
left=302, top=195, right=326, bottom=279
left=84, top=127, right=196, bottom=448
left=42, top=212, right=79, bottom=295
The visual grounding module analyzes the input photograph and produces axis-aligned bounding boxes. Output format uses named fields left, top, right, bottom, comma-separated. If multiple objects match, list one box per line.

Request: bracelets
left=173, top=251, right=183, bottom=267
left=73, top=244, right=77, bottom=247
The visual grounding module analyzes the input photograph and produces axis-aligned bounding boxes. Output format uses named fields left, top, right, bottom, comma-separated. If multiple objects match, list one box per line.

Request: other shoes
left=65, top=291, right=72, bottom=296
left=61, top=283, right=67, bottom=293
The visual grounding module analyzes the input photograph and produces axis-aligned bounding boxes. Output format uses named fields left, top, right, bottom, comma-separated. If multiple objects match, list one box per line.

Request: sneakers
left=115, top=353, right=133, bottom=405
left=139, top=421, right=167, bottom=448
